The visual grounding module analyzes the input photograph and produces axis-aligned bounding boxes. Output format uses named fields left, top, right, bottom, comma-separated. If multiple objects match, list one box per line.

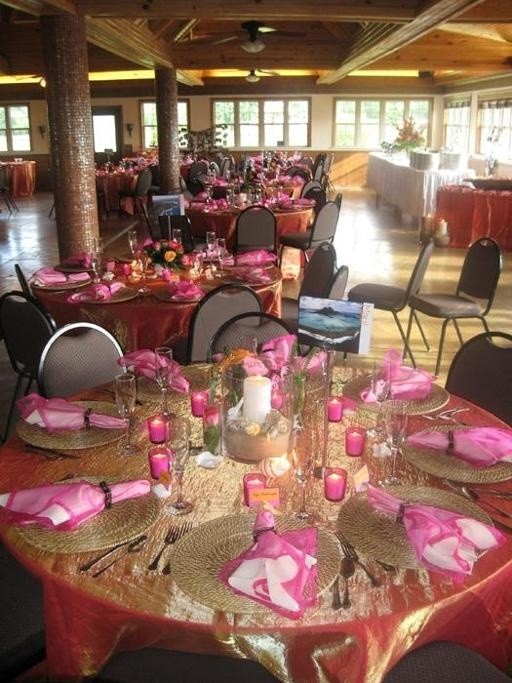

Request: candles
left=327, top=396, right=344, bottom=422
left=345, top=426, right=365, bottom=457
left=148, top=447, right=169, bottom=480
left=243, top=372, right=271, bottom=426
left=243, top=473, right=268, bottom=509
left=191, top=392, right=209, bottom=417
left=323, top=467, right=348, bottom=502
left=147, top=415, right=165, bottom=443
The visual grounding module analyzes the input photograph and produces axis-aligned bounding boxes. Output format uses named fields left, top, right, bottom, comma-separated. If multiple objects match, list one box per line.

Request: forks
left=148, top=520, right=193, bottom=577
left=338, top=529, right=379, bottom=588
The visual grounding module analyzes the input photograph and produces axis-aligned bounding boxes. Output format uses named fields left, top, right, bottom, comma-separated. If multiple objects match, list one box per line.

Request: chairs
left=2, top=152, right=57, bottom=222
left=1, top=289, right=59, bottom=447
left=443, top=331, right=511, bottom=430
left=401, top=237, right=502, bottom=375
left=79, top=644, right=284, bottom=681
left=16, top=262, right=35, bottom=305
left=348, top=230, right=430, bottom=369
left=165, top=282, right=264, bottom=364
left=207, top=310, right=302, bottom=361
left=95, top=153, right=344, bottom=278
left=37, top=322, right=128, bottom=403
left=281, top=265, right=350, bottom=358
left=280, top=242, right=338, bottom=319
left=3, top=549, right=57, bottom=676
left=380, top=637, right=511, bottom=682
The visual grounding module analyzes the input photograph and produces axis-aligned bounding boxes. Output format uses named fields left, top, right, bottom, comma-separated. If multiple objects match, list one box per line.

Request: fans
left=210, top=21, right=306, bottom=46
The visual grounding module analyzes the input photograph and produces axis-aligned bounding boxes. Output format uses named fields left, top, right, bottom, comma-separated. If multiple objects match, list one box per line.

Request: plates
left=342, top=371, right=452, bottom=415
left=152, top=288, right=206, bottom=303
left=14, top=474, right=163, bottom=553
left=24, top=258, right=102, bottom=292
left=409, top=148, right=466, bottom=171
left=71, top=285, right=135, bottom=303
left=12, top=398, right=130, bottom=449
left=224, top=259, right=277, bottom=271
left=221, top=272, right=282, bottom=287
left=168, top=512, right=344, bottom=616
left=400, top=422, right=512, bottom=486
left=341, top=482, right=495, bottom=571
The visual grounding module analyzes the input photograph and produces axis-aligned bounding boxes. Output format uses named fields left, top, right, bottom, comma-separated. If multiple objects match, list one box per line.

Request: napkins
left=171, top=281, right=201, bottom=299
left=2, top=477, right=151, bottom=532
left=259, top=333, right=329, bottom=378
left=359, top=350, right=438, bottom=405
left=116, top=348, right=189, bottom=393
left=14, top=391, right=130, bottom=431
left=220, top=502, right=319, bottom=623
left=74, top=280, right=125, bottom=304
left=406, top=427, right=510, bottom=469
left=363, top=482, right=508, bottom=578
left=35, top=269, right=90, bottom=288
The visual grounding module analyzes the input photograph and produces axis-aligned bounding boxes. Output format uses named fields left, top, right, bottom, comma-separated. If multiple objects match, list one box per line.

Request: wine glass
left=83, top=228, right=227, bottom=292
left=94, top=148, right=194, bottom=174
left=203, top=146, right=303, bottom=212
left=106, top=342, right=411, bottom=520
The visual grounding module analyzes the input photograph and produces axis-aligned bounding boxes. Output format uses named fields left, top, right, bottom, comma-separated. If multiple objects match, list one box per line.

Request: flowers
left=204, top=344, right=307, bottom=452
left=380, top=111, right=428, bottom=156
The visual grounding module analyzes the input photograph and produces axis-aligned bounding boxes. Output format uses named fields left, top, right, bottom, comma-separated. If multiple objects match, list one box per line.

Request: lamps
left=245, top=67, right=261, bottom=83
left=242, top=27, right=266, bottom=55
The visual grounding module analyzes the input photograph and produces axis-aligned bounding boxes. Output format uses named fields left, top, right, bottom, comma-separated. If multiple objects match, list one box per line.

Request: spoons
left=459, top=483, right=511, bottom=520
left=92, top=535, right=145, bottom=579
left=340, top=556, right=355, bottom=609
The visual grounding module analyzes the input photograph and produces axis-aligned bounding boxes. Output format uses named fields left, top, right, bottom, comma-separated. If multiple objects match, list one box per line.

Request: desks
left=361, top=153, right=512, bottom=252
